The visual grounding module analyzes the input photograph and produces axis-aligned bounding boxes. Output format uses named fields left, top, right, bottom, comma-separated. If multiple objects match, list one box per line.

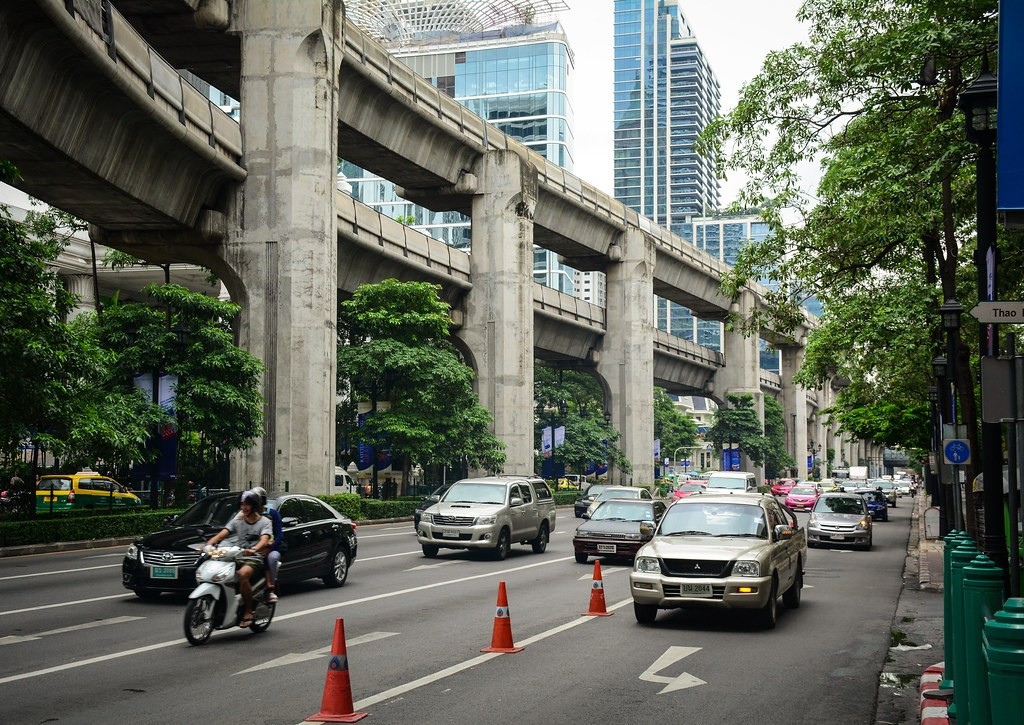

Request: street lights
left=542, top=399, right=569, bottom=480
left=955, top=40, right=1008, bottom=576
left=930, top=351, right=954, bottom=542
left=933, top=295, right=967, bottom=531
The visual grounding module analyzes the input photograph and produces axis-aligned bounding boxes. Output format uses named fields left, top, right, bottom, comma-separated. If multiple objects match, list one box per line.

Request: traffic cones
left=577, top=557, right=618, bottom=618
left=302, top=615, right=371, bottom=723
left=480, top=581, right=524, bottom=653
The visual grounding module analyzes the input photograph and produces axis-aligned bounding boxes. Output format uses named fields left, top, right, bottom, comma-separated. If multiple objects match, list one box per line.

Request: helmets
left=252, top=487, right=268, bottom=505
left=237, top=491, right=259, bottom=512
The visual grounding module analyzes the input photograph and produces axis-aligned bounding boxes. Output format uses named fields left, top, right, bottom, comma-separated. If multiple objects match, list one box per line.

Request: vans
left=334, top=465, right=361, bottom=497
left=699, top=471, right=758, bottom=492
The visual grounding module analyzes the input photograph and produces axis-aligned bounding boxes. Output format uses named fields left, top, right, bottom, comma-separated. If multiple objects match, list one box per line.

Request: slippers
left=239, top=617, right=254, bottom=628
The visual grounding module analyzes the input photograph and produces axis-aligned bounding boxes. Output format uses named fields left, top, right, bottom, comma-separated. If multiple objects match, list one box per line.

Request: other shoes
left=265, top=585, right=278, bottom=602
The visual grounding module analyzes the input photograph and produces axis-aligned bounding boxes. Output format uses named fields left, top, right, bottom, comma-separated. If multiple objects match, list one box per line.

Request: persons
left=357, top=483, right=372, bottom=497
left=840, top=486, right=847, bottom=493
left=252, top=487, right=282, bottom=601
left=382, top=478, right=398, bottom=496
left=198, top=490, right=273, bottom=629
left=909, top=481, right=916, bottom=488
left=9, top=470, right=24, bottom=494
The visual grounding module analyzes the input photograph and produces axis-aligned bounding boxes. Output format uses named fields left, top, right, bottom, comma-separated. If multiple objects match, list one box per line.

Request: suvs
left=416, top=474, right=557, bottom=561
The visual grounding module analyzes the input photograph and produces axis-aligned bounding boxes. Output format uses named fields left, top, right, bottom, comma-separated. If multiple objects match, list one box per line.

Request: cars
left=671, top=470, right=715, bottom=502
left=784, top=484, right=820, bottom=510
left=413, top=481, right=454, bottom=532
left=549, top=473, right=589, bottom=489
left=33, top=471, right=144, bottom=513
left=770, top=478, right=798, bottom=496
left=572, top=483, right=613, bottom=518
left=121, top=491, right=359, bottom=601
left=853, top=489, right=888, bottom=522
left=805, top=492, right=874, bottom=549
left=584, top=484, right=661, bottom=519
left=572, top=497, right=668, bottom=566
left=817, top=466, right=918, bottom=507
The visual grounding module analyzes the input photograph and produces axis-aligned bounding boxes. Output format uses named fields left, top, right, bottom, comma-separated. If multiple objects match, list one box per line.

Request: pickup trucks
left=630, top=491, right=809, bottom=631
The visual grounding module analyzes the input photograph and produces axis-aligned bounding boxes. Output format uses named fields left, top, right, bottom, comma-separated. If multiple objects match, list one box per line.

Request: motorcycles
left=183, top=541, right=281, bottom=646
